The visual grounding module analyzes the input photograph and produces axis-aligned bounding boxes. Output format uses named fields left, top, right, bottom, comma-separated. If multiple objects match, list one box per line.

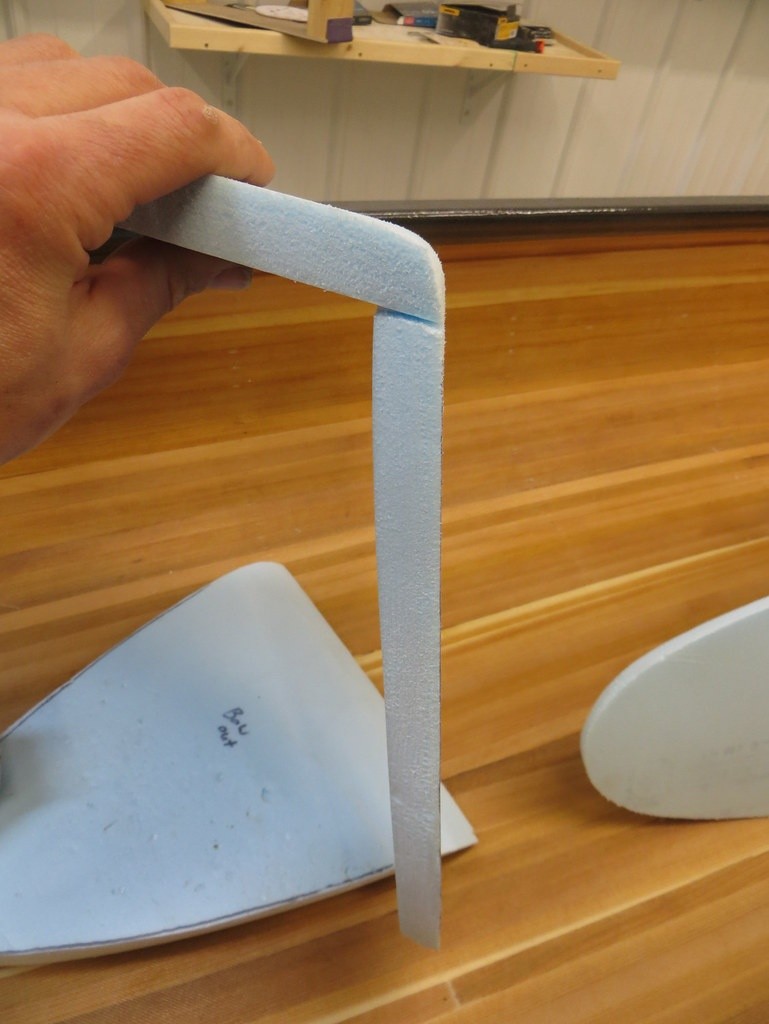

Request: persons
left=0, top=32, right=274, bottom=461
